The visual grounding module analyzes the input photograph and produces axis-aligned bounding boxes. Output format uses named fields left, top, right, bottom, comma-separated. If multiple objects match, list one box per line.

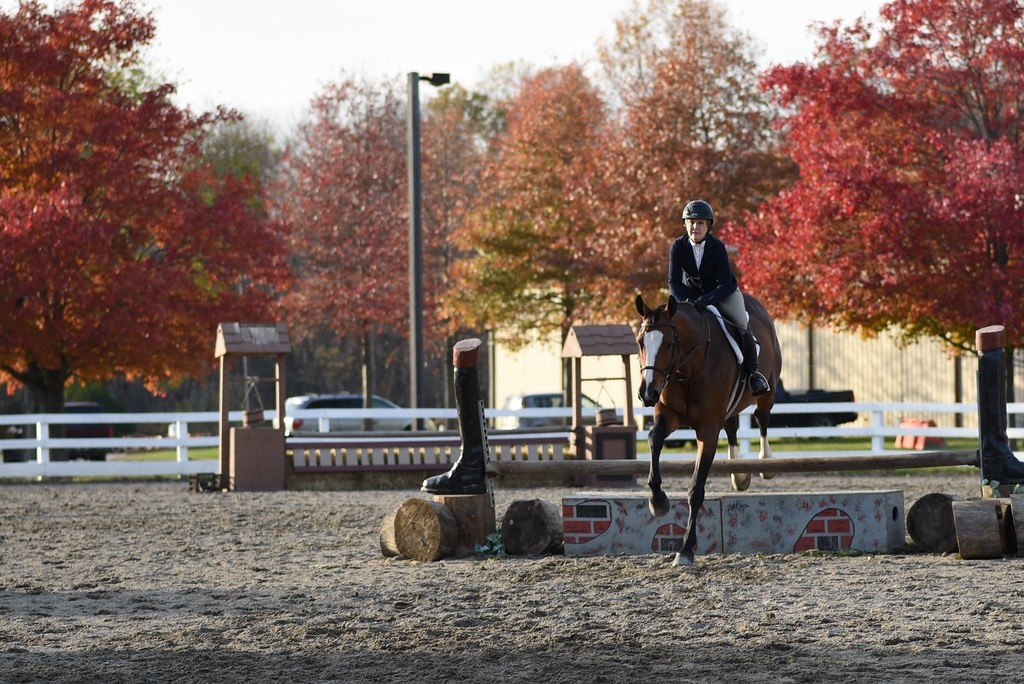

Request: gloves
left=694, top=296, right=707, bottom=311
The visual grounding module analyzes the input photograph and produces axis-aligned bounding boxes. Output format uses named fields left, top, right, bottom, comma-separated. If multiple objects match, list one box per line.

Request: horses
left=633, top=291, right=782, bottom=571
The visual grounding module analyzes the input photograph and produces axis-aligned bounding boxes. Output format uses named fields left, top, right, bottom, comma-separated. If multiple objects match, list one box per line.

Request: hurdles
left=420, top=325, right=1024, bottom=562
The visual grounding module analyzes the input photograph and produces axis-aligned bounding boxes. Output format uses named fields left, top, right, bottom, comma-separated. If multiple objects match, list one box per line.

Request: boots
left=421, top=366, right=486, bottom=494
left=734, top=323, right=767, bottom=396
left=975, top=348, right=1024, bottom=484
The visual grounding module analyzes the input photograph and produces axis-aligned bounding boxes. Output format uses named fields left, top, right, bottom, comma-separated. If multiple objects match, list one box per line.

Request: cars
left=516, top=391, right=639, bottom=440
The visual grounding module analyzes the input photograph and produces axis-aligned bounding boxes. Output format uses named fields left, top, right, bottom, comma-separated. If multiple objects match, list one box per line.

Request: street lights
left=406, top=69, right=452, bottom=431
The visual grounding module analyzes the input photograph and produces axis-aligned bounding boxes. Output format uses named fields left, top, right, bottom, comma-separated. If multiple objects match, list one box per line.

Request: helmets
left=682, top=200, right=714, bottom=225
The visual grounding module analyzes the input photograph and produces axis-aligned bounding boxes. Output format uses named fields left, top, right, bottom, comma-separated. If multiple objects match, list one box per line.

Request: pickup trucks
left=662, top=376, right=858, bottom=449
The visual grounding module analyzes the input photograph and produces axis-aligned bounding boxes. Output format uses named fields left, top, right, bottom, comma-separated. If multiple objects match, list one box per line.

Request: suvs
left=284, top=390, right=439, bottom=439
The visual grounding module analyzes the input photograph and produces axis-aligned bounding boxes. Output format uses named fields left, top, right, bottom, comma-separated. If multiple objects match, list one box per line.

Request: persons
left=668, top=200, right=770, bottom=397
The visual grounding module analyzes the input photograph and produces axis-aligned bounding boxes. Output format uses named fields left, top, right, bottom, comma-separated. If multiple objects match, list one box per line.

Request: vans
left=63, top=401, right=113, bottom=461
